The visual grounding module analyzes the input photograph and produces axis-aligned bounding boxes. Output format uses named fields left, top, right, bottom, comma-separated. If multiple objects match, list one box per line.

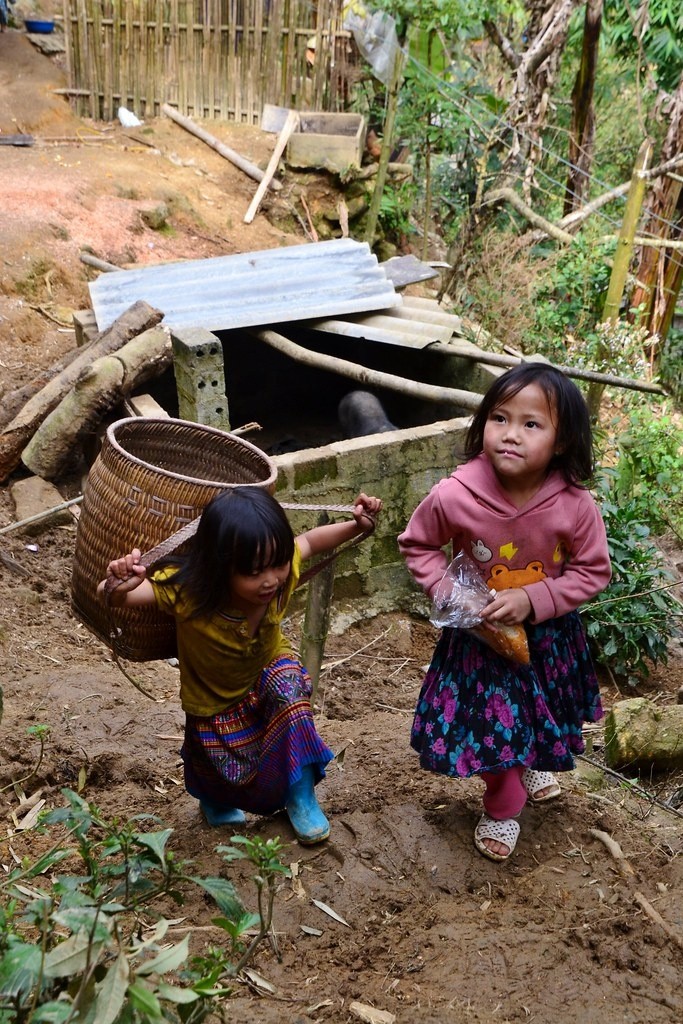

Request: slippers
left=474, top=811, right=520, bottom=861
left=521, top=766, right=562, bottom=801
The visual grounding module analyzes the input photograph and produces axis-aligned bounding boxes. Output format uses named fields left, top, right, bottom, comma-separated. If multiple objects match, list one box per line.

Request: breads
left=478, top=620, right=530, bottom=664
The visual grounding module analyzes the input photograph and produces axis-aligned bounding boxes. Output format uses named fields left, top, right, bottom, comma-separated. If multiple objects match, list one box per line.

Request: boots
left=286, top=765, right=330, bottom=844
left=200, top=791, right=246, bottom=828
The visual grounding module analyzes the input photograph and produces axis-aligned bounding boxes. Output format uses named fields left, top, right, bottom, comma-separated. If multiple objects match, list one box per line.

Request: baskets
left=69, top=416, right=275, bottom=661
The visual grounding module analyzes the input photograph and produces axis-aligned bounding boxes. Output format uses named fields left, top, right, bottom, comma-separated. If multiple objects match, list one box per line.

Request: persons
left=397, top=364, right=612, bottom=860
left=97, top=487, right=383, bottom=845
